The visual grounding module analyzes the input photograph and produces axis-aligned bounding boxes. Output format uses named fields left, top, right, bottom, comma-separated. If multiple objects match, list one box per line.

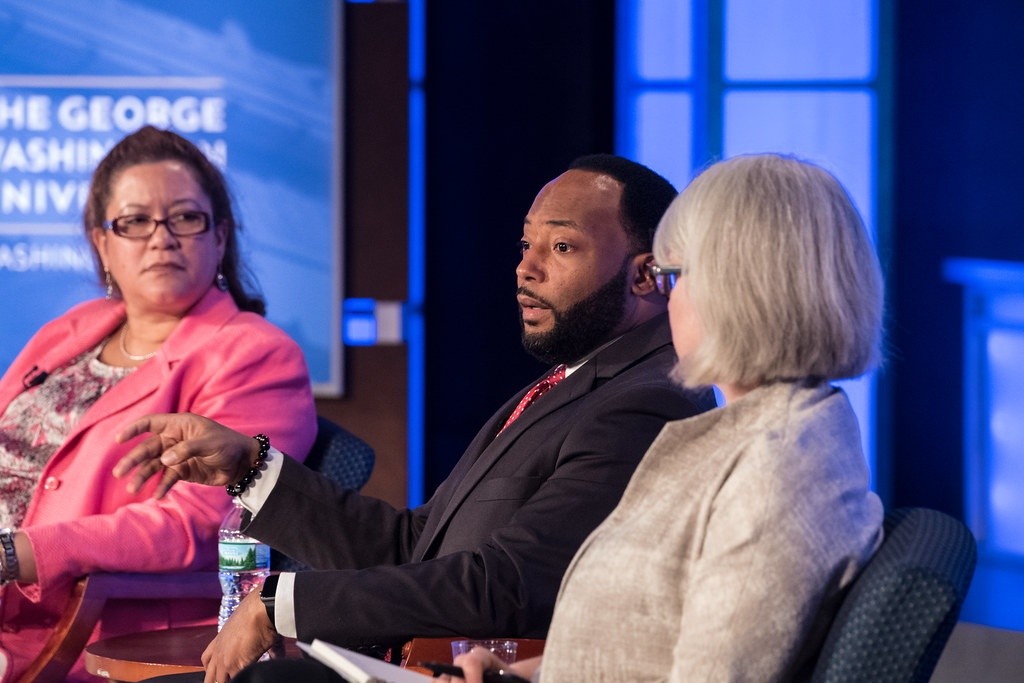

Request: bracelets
left=0, top=528, right=21, bottom=584
left=226, top=434, right=270, bottom=497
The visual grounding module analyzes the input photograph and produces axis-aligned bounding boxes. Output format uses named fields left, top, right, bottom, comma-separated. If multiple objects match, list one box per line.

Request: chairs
left=395, top=503, right=979, bottom=683
left=15, top=413, right=377, bottom=683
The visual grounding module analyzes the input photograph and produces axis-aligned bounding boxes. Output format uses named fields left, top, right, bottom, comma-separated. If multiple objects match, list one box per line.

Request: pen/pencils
left=415, top=661, right=533, bottom=683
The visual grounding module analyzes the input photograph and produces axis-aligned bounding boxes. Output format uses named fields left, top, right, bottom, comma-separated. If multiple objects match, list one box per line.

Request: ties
left=497, top=364, right=566, bottom=436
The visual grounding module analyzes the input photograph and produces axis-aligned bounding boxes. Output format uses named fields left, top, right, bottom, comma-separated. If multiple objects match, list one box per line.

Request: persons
left=111, top=157, right=719, bottom=683
left=438, top=150, right=884, bottom=683
left=0, top=125, right=318, bottom=683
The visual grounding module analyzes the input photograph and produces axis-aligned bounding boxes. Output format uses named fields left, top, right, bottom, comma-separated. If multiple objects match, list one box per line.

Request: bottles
left=217, top=496, right=272, bottom=662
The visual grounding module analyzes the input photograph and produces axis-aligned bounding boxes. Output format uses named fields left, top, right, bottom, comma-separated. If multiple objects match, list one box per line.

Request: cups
left=451, top=641, right=518, bottom=665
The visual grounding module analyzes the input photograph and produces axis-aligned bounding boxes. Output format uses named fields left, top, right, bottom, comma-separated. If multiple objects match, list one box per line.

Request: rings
left=215, top=682, right=218, bottom=683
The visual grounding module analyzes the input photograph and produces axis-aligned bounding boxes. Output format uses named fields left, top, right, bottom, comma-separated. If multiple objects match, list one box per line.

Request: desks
left=83, top=623, right=304, bottom=683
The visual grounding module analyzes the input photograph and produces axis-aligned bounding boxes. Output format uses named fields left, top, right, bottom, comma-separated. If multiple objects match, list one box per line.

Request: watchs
left=259, top=573, right=281, bottom=631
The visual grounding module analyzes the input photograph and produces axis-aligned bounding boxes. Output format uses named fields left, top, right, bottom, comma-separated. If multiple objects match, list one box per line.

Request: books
left=295, top=638, right=449, bottom=683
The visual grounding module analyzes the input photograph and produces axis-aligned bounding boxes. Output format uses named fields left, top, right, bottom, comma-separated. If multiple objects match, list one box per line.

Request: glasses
left=653, top=263, right=683, bottom=297
left=102, top=208, right=220, bottom=239
